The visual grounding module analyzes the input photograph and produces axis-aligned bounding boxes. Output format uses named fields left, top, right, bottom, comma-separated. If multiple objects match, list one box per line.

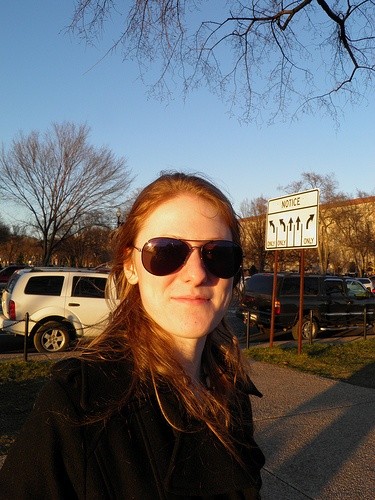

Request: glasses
left=129, top=237, right=243, bottom=279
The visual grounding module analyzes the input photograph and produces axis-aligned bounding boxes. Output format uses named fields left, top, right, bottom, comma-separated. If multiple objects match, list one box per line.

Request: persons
left=0, top=167, right=270, bottom=500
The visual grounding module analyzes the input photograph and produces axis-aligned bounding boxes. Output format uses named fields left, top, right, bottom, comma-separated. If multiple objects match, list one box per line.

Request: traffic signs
left=264, top=189, right=320, bottom=250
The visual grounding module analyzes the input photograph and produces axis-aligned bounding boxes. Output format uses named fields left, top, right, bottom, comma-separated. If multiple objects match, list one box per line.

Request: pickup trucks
left=0, top=268, right=123, bottom=353
left=234, top=272, right=375, bottom=340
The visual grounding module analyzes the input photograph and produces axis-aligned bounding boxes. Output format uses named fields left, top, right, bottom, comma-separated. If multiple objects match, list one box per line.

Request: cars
left=355, top=277, right=374, bottom=293
left=0, top=264, right=29, bottom=289
